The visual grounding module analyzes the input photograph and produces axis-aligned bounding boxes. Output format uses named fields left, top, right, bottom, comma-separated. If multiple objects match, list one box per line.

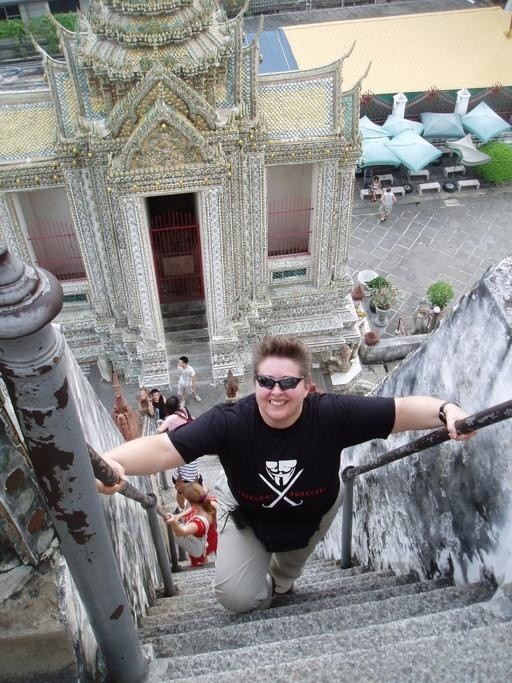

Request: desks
left=357, top=269, right=378, bottom=297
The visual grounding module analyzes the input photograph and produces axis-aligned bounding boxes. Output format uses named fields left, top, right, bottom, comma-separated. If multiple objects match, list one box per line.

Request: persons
left=377, top=188, right=397, bottom=221
left=141, top=355, right=221, bottom=567
left=368, top=176, right=383, bottom=202
left=95, top=333, right=478, bottom=614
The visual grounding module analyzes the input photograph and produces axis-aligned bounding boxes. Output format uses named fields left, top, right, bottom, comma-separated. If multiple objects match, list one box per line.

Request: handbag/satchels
left=175, top=478, right=199, bottom=494
left=174, top=516, right=209, bottom=558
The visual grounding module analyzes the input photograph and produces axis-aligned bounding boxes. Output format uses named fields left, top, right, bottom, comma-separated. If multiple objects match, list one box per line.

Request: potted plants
left=372, top=285, right=399, bottom=327
left=364, top=275, right=392, bottom=313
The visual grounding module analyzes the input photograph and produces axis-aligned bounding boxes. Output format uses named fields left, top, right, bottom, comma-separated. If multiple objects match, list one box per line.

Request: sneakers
left=181, top=400, right=185, bottom=406
left=193, top=394, right=201, bottom=402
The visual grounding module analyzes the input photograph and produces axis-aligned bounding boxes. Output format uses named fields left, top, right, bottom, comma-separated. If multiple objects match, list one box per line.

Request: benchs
left=373, top=174, right=393, bottom=185
left=359, top=189, right=383, bottom=201
left=457, top=178, right=480, bottom=191
left=424, top=134, right=504, bottom=158
left=443, top=165, right=466, bottom=178
left=383, top=186, right=406, bottom=197
left=408, top=170, right=430, bottom=181
left=417, top=182, right=441, bottom=195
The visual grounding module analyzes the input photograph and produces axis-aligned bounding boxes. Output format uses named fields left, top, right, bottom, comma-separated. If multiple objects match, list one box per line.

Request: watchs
left=438, top=402, right=460, bottom=424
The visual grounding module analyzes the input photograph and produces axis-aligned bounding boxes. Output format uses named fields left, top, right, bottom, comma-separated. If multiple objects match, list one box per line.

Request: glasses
left=256, top=375, right=305, bottom=390
